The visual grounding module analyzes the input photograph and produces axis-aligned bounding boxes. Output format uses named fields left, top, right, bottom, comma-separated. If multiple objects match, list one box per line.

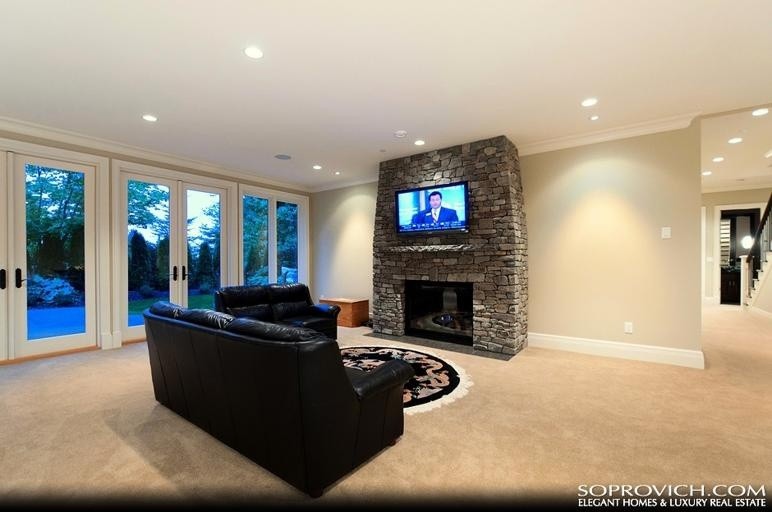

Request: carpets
left=337, top=343, right=476, bottom=415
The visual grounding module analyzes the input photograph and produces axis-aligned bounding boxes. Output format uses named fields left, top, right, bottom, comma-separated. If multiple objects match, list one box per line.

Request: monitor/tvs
left=395, top=182, right=469, bottom=234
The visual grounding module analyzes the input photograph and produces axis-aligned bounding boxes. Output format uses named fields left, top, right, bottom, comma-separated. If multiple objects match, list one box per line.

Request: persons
left=417, top=191, right=458, bottom=223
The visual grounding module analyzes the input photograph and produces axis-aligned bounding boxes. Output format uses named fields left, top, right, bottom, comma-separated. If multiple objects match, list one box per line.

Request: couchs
left=142, top=281, right=415, bottom=500
